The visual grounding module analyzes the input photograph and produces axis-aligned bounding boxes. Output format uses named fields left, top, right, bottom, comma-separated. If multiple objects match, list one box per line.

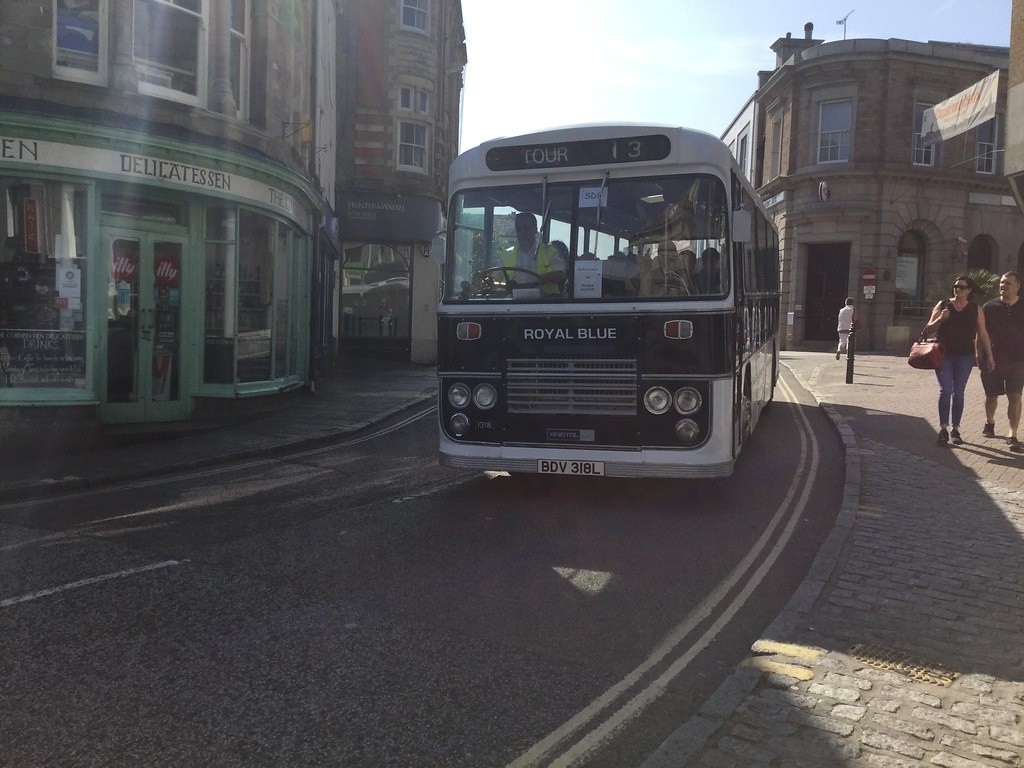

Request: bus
left=439, top=123, right=784, bottom=480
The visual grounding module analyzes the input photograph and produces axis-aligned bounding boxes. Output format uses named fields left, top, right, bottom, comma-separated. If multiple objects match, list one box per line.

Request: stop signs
left=862, top=269, right=877, bottom=286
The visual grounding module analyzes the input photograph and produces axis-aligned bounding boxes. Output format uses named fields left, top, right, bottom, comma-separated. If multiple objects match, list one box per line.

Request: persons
left=927, top=276, right=995, bottom=444
left=471, top=213, right=566, bottom=296
left=836, top=297, right=857, bottom=360
left=376, top=297, right=392, bottom=328
left=552, top=239, right=725, bottom=297
left=975, top=271, right=1024, bottom=451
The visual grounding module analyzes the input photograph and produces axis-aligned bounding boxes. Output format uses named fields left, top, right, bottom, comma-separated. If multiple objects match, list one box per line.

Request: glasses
left=515, top=223, right=536, bottom=232
left=953, top=285, right=970, bottom=290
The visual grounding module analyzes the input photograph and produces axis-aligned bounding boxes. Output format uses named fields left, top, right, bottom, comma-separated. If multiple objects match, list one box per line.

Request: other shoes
left=938, top=429, right=949, bottom=442
left=1007, top=437, right=1020, bottom=450
left=836, top=350, right=840, bottom=360
left=951, top=429, right=962, bottom=444
left=982, top=423, right=994, bottom=437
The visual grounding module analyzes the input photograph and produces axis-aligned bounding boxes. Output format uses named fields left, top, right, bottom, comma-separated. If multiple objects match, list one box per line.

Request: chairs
left=600, top=259, right=703, bottom=298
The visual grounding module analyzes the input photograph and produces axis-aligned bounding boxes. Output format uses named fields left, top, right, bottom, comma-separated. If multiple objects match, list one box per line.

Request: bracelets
left=987, top=358, right=994, bottom=359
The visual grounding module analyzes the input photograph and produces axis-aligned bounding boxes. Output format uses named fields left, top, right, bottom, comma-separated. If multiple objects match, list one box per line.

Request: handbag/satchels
left=908, top=325, right=945, bottom=369
